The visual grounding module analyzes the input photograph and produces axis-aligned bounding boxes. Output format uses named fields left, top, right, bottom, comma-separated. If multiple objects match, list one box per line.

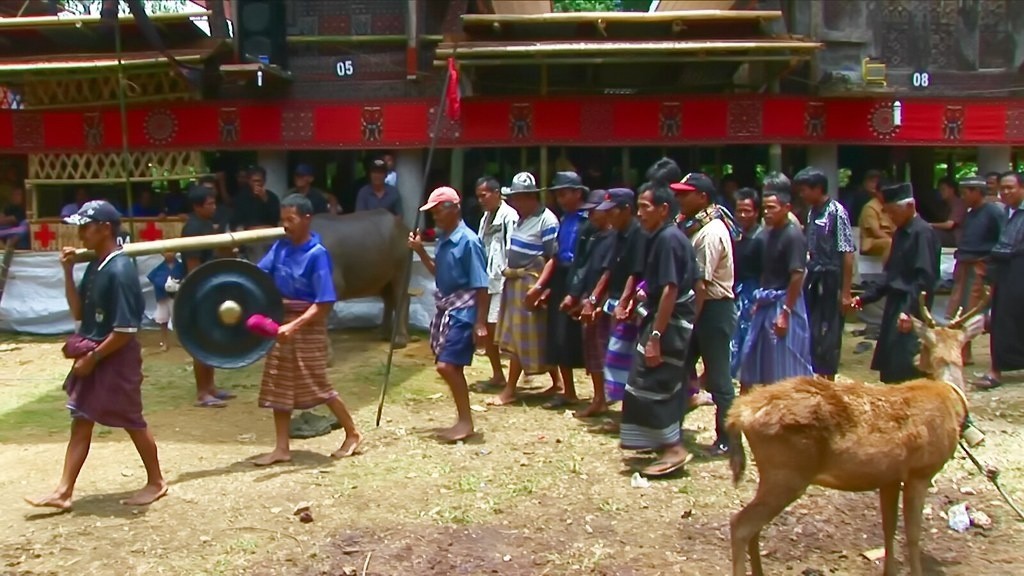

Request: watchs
left=589, top=296, right=600, bottom=306
left=651, top=329, right=662, bottom=339
left=91, top=348, right=101, bottom=363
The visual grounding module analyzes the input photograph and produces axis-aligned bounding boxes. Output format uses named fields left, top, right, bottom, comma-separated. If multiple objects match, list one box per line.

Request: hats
left=293, top=162, right=314, bottom=176
left=501, top=172, right=547, bottom=194
left=671, top=173, right=715, bottom=195
left=418, top=186, right=461, bottom=211
left=595, top=189, right=634, bottom=209
left=580, top=190, right=605, bottom=210
left=370, top=159, right=388, bottom=170
left=884, top=184, right=912, bottom=203
left=959, top=176, right=986, bottom=190
left=550, top=172, right=591, bottom=193
left=61, top=200, right=122, bottom=225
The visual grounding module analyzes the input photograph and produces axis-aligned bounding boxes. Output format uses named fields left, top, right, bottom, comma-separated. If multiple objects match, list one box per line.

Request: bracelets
left=534, top=284, right=543, bottom=291
left=783, top=305, right=791, bottom=315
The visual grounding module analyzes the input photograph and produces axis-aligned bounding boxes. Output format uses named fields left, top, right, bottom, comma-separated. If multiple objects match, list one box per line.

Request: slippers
left=974, top=374, right=1003, bottom=388
left=853, top=341, right=873, bottom=354
left=705, top=444, right=731, bottom=458
left=546, top=396, right=578, bottom=410
left=194, top=391, right=238, bottom=408
left=642, top=453, right=693, bottom=475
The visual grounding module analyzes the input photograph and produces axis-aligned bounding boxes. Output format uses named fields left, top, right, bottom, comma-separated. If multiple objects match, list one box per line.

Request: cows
left=250, top=210, right=417, bottom=347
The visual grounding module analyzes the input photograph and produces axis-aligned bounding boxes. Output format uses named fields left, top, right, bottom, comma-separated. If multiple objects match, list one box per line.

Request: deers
left=724, top=283, right=992, bottom=576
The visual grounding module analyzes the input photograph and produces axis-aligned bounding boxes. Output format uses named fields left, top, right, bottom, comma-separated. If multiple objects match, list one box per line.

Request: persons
left=408, top=185, right=492, bottom=442
left=179, top=186, right=238, bottom=408
left=249, top=193, right=363, bottom=466
left=1, top=152, right=403, bottom=252
left=472, top=156, right=1024, bottom=476
left=23, top=200, right=169, bottom=517
left=146, top=249, right=185, bottom=351
